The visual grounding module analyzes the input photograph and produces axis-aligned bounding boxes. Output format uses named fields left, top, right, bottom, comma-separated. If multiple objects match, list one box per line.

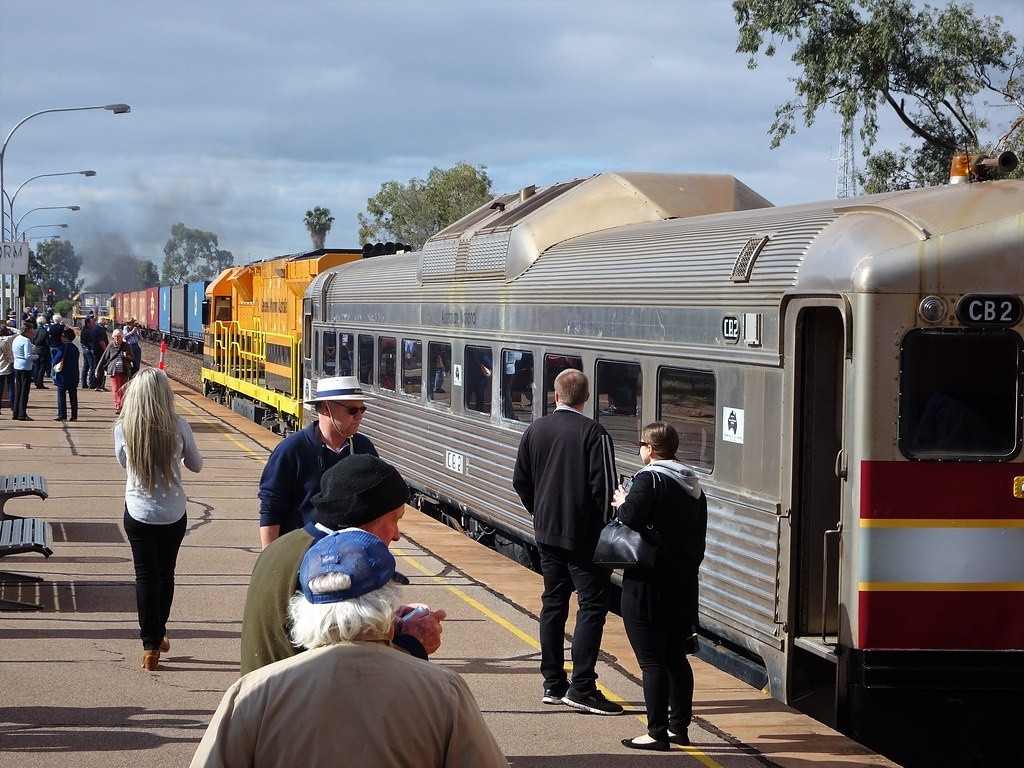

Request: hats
left=299, top=529, right=396, bottom=604
left=8, top=311, right=16, bottom=317
left=311, top=454, right=408, bottom=530
left=125, top=317, right=136, bottom=322
left=22, top=313, right=27, bottom=319
left=304, top=374, right=378, bottom=404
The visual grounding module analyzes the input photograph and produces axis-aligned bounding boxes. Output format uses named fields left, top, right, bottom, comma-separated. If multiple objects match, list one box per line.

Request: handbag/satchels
left=52, top=343, right=66, bottom=372
left=593, top=480, right=641, bottom=569
left=103, top=361, right=110, bottom=369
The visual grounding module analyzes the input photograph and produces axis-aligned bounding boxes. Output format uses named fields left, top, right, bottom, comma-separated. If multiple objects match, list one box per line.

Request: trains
left=81, top=280, right=213, bottom=353
left=201, top=172, right=1024, bottom=732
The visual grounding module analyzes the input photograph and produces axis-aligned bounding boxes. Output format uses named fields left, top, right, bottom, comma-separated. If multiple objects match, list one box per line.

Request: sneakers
left=561, top=686, right=624, bottom=716
left=540, top=680, right=572, bottom=703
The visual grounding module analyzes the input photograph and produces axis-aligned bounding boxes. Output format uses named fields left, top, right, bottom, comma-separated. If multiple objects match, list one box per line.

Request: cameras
left=400, top=606, right=434, bottom=622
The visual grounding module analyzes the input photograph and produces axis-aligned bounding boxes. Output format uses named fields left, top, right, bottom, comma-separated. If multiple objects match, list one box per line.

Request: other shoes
left=37, top=385, right=49, bottom=389
left=670, top=734, right=690, bottom=746
left=621, top=737, right=671, bottom=752
left=11, top=413, right=19, bottom=419
left=19, top=415, right=34, bottom=421
left=54, top=417, right=66, bottom=421
left=159, top=637, right=170, bottom=652
left=70, top=417, right=77, bottom=421
left=84, top=386, right=107, bottom=391
left=115, top=410, right=120, bottom=413
left=140, top=650, right=160, bottom=670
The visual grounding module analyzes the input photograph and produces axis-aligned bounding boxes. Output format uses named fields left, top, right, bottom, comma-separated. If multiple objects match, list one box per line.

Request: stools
left=0, top=473, right=49, bottom=524
left=0, top=518, right=55, bottom=613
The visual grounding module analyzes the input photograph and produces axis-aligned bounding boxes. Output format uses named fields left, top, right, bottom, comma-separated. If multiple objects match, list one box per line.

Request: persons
left=611, top=423, right=708, bottom=751
left=512, top=368, right=625, bottom=715
left=1, top=306, right=143, bottom=421
left=115, top=367, right=204, bottom=672
left=258, top=376, right=379, bottom=552
left=240, top=455, right=448, bottom=678
left=323, top=332, right=638, bottom=421
left=189, top=527, right=510, bottom=768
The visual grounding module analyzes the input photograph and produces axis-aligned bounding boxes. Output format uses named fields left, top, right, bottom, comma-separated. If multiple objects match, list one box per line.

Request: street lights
left=0, top=104, right=131, bottom=324
left=3, top=170, right=96, bottom=315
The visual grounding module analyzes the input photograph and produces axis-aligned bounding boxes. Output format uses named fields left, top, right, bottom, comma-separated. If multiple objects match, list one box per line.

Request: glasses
left=638, top=439, right=648, bottom=447
left=331, top=399, right=368, bottom=415
